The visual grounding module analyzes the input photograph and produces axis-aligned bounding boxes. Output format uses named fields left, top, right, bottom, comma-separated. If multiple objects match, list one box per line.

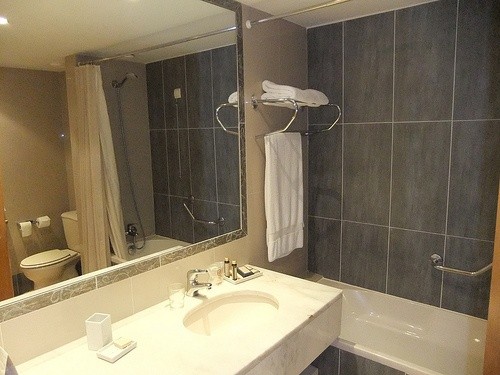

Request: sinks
left=183, top=289, right=280, bottom=338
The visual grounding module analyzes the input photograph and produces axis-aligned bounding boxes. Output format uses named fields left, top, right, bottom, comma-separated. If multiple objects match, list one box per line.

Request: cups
left=86, top=312, right=112, bottom=352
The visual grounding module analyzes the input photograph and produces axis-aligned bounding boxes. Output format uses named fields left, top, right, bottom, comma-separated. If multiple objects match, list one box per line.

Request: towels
left=260, top=78, right=329, bottom=110
left=263, top=132, right=305, bottom=263
left=228, top=91, right=239, bottom=109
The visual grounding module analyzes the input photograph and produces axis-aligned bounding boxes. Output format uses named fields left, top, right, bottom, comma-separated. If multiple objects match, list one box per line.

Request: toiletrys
left=232, top=260, right=238, bottom=280
left=223, top=258, right=230, bottom=277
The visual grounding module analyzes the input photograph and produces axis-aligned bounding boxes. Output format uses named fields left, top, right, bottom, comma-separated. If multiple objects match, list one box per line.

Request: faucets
left=186, top=269, right=212, bottom=297
left=128, top=222, right=138, bottom=238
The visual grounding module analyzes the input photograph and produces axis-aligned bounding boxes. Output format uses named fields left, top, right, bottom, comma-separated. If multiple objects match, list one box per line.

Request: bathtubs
left=311, top=271, right=488, bottom=375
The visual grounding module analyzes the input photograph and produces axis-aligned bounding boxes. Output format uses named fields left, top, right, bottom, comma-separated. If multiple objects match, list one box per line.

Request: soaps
left=114, top=337, right=132, bottom=349
left=238, top=265, right=255, bottom=279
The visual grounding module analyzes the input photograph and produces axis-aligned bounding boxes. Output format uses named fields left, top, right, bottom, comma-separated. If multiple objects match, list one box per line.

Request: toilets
left=19, top=207, right=81, bottom=291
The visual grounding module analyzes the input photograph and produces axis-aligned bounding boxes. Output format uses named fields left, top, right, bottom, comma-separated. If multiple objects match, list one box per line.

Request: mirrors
left=0, top=0, right=247, bottom=321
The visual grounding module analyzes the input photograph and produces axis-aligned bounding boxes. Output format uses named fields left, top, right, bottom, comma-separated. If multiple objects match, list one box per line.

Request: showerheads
left=116, top=70, right=138, bottom=86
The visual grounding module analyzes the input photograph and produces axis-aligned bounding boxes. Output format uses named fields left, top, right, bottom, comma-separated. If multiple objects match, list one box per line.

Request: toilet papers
left=18, top=221, right=32, bottom=238
left=36, top=215, right=51, bottom=228
